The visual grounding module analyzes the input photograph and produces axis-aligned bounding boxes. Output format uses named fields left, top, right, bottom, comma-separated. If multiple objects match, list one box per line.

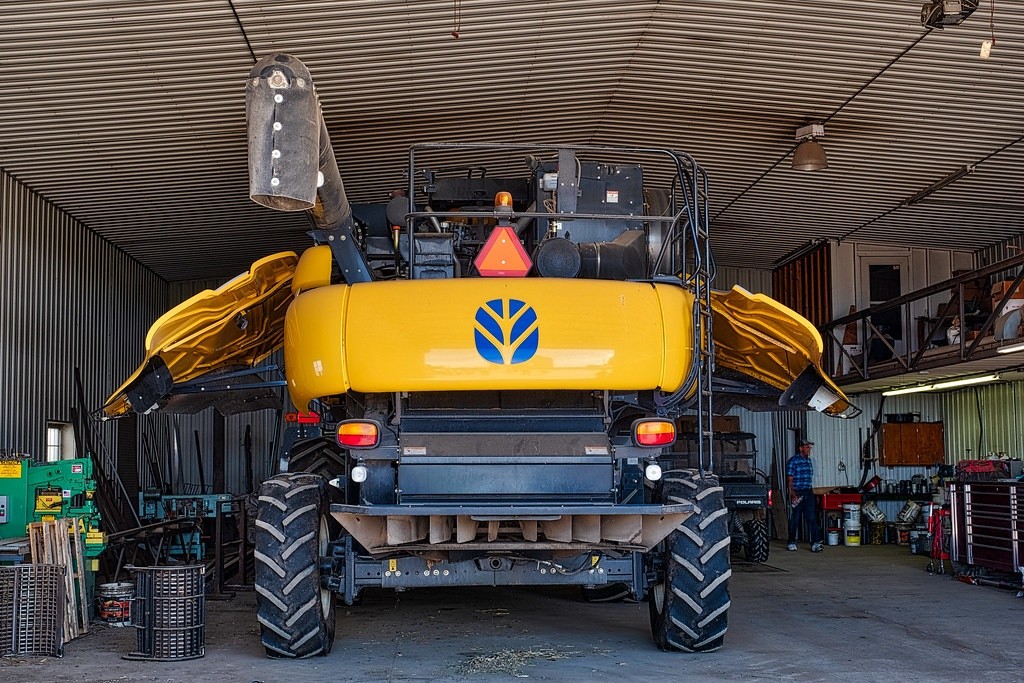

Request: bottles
left=829, top=531, right=838, bottom=545
left=836, top=515, right=841, bottom=528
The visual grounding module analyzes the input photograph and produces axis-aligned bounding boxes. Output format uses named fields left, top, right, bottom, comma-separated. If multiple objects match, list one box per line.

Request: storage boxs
left=991, top=280, right=1024, bottom=301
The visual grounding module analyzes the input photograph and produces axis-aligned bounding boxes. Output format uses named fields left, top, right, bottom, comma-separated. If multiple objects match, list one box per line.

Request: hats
left=799, top=440, right=814, bottom=446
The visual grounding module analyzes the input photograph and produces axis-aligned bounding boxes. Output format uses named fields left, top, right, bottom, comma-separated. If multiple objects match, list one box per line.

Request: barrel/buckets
left=887, top=522, right=895, bottom=544
left=870, top=522, right=887, bottom=544
left=909, top=528, right=929, bottom=553
left=897, top=502, right=921, bottom=521
left=915, top=524, right=925, bottom=528
left=99, top=582, right=135, bottom=627
left=843, top=504, right=861, bottom=526
left=862, top=501, right=886, bottom=522
left=895, top=523, right=914, bottom=545
left=844, top=526, right=861, bottom=547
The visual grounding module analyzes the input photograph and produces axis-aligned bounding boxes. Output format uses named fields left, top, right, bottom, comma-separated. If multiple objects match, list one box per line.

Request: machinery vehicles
left=88, top=52, right=862, bottom=663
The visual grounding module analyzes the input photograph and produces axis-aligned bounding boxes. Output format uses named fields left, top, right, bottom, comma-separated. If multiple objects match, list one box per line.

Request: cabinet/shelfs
left=878, top=422, right=945, bottom=466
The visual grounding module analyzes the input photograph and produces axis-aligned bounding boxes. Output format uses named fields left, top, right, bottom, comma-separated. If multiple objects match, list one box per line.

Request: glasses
left=802, top=446, right=812, bottom=449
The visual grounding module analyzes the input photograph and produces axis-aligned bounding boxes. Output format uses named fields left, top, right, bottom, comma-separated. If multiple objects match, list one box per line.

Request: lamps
left=979, top=0, right=998, bottom=61
left=792, top=123, right=828, bottom=173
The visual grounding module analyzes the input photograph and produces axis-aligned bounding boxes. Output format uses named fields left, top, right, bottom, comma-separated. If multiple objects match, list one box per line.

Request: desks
left=946, top=479, right=1024, bottom=574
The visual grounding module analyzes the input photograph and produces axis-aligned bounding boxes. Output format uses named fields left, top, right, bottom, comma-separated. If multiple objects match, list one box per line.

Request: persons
left=787, top=439, right=824, bottom=553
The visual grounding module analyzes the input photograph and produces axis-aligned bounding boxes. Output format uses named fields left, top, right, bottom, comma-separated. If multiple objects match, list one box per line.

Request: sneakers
left=812, top=543, right=824, bottom=552
left=786, top=542, right=797, bottom=551
